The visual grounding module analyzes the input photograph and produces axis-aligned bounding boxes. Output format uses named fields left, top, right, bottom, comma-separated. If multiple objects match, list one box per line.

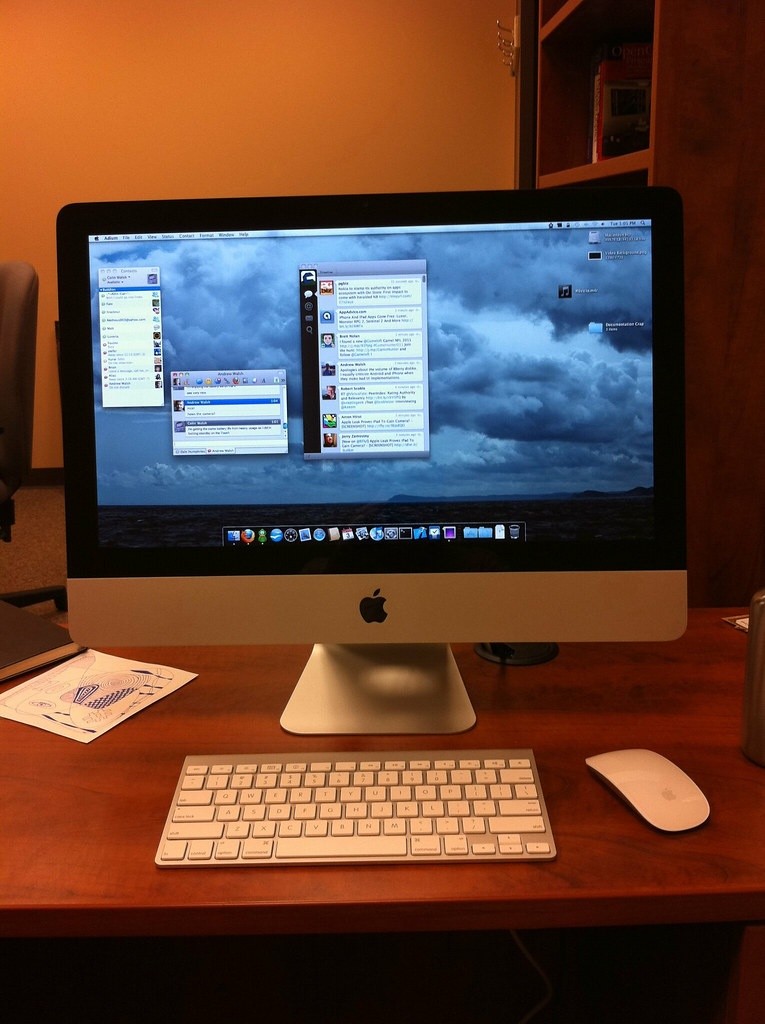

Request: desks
left=0, top=605, right=765, bottom=1024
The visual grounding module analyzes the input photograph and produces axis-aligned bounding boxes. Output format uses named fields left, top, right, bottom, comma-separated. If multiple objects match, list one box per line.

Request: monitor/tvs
left=54, top=186, right=691, bottom=648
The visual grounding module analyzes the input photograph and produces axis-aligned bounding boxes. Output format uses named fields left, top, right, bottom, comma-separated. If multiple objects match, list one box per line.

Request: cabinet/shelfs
left=507, top=1, right=765, bottom=605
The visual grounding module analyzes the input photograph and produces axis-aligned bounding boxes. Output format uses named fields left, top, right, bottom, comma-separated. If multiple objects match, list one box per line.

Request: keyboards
left=154, top=748, right=558, bottom=866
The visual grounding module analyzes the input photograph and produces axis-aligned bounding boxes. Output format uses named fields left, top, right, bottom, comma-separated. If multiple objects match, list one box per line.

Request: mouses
left=585, top=750, right=710, bottom=832
